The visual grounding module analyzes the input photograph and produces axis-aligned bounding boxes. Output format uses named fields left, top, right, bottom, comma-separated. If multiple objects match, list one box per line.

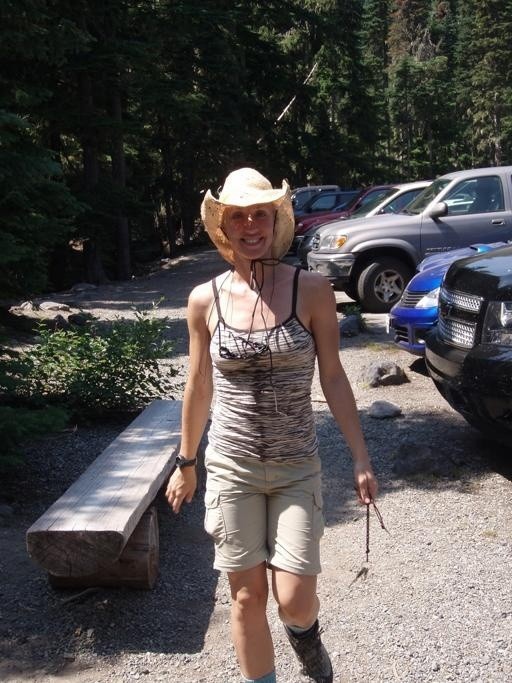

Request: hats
left=202, top=168, right=296, bottom=266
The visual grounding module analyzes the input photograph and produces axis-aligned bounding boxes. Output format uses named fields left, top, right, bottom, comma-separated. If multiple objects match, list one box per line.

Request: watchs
left=175, top=455, right=196, bottom=468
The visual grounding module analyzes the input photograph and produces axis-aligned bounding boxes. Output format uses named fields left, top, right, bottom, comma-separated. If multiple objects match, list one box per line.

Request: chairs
left=469, top=178, right=504, bottom=211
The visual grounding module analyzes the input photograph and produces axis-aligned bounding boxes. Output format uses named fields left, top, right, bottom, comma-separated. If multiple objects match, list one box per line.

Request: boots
left=284, top=620, right=333, bottom=682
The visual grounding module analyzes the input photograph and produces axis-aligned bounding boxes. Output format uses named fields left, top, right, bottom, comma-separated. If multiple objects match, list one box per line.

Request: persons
left=164, top=167, right=378, bottom=683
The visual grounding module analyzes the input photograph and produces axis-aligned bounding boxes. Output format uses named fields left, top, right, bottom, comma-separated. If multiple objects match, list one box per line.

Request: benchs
left=26, top=399, right=183, bottom=589
